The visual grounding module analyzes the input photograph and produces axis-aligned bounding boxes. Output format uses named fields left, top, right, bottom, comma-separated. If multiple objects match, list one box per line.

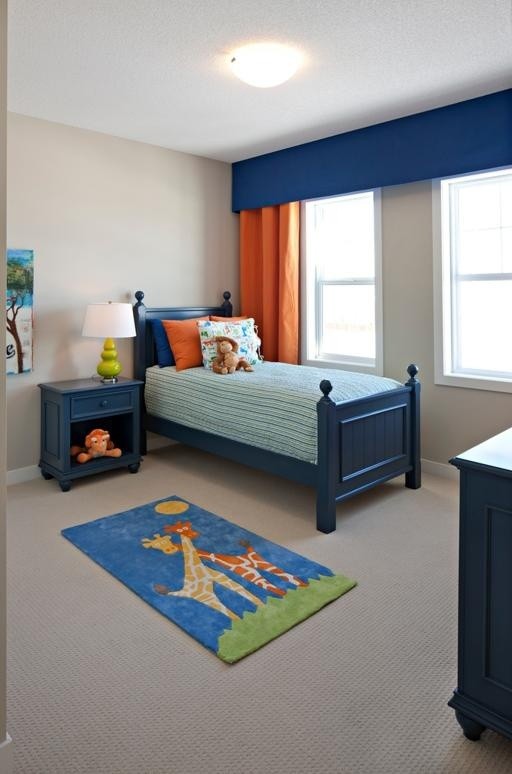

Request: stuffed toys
left=211, top=335, right=256, bottom=375
left=70, top=427, right=123, bottom=464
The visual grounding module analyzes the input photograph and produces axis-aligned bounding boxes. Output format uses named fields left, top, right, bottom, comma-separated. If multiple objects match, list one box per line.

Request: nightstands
left=37, top=376, right=144, bottom=492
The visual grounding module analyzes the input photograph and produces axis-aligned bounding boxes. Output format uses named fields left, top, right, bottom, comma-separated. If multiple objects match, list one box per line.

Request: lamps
left=81, top=302, right=137, bottom=384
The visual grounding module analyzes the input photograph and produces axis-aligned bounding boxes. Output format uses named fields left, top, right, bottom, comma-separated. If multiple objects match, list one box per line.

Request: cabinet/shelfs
left=447, top=427, right=512, bottom=741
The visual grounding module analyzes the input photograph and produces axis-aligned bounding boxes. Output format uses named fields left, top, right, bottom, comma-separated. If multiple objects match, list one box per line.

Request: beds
left=133, top=291, right=421, bottom=534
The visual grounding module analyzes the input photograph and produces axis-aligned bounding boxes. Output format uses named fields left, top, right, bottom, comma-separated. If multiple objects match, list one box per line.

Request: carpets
left=61, top=494, right=358, bottom=664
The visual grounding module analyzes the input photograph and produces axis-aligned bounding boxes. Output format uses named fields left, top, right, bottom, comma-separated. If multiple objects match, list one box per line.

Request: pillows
left=150, top=315, right=258, bottom=372
